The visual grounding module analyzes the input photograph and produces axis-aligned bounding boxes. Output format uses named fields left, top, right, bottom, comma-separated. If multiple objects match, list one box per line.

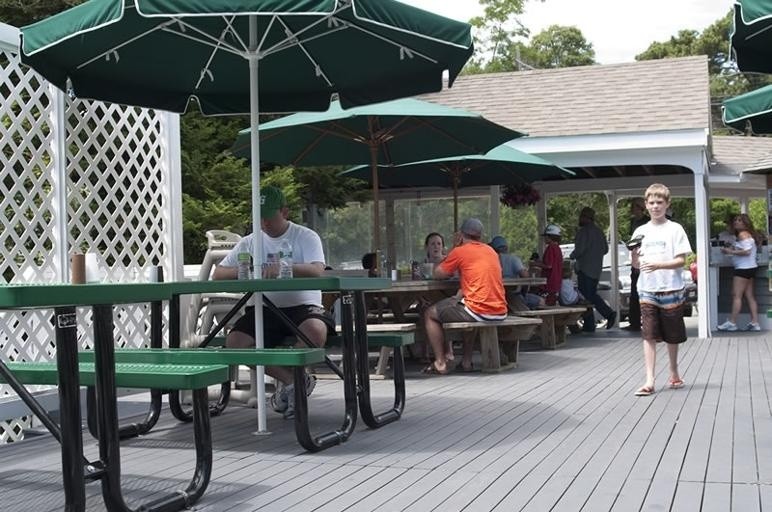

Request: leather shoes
left=606, top=311, right=617, bottom=329
left=620, top=325, right=641, bottom=331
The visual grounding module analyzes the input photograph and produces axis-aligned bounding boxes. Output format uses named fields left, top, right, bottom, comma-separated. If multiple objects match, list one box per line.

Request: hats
left=631, top=197, right=646, bottom=210
left=580, top=206, right=595, bottom=218
left=529, top=252, right=539, bottom=260
left=249, top=185, right=286, bottom=222
left=540, top=224, right=562, bottom=237
left=459, top=217, right=484, bottom=238
left=491, top=236, right=507, bottom=250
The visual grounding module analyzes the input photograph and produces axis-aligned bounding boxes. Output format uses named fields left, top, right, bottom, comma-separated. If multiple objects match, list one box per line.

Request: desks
left=167, top=277, right=393, bottom=452
left=0, top=276, right=173, bottom=512
left=310, top=279, right=461, bottom=382
left=502, top=276, right=548, bottom=287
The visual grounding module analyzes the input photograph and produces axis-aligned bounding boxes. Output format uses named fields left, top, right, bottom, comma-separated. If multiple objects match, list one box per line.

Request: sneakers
left=270, top=374, right=317, bottom=420
left=716, top=321, right=738, bottom=331
left=740, top=321, right=761, bottom=331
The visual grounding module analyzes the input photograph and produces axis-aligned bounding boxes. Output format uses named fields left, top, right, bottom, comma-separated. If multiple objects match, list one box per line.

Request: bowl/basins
left=626, top=240, right=641, bottom=250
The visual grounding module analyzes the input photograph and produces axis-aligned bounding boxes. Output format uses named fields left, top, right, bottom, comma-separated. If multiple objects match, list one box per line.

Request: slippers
left=634, top=384, right=657, bottom=395
left=421, top=362, right=449, bottom=375
left=668, top=378, right=685, bottom=388
left=454, top=361, right=474, bottom=373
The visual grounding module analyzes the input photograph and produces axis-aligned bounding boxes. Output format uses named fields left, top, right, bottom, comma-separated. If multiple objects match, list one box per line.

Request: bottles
left=376, top=248, right=382, bottom=271
left=380, top=255, right=387, bottom=278
left=279, top=239, right=293, bottom=280
left=157, top=266, right=164, bottom=281
left=239, top=239, right=251, bottom=280
left=152, top=266, right=157, bottom=281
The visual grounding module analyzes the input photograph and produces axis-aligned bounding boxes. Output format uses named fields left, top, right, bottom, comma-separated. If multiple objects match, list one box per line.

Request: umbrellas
left=236, top=98, right=529, bottom=324
left=18, top=0, right=474, bottom=436
left=728, top=0, right=772, bottom=76
left=334, top=144, right=576, bottom=248
left=721, top=84, right=772, bottom=135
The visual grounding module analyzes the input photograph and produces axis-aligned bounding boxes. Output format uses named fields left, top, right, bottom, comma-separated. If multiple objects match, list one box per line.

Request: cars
left=559, top=241, right=698, bottom=329
left=339, top=261, right=363, bottom=270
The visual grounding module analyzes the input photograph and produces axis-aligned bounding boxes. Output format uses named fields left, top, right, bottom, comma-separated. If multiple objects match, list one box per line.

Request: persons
left=212, top=185, right=328, bottom=420
left=715, top=213, right=760, bottom=332
left=569, top=207, right=617, bottom=333
left=620, top=198, right=651, bottom=331
left=528, top=225, right=563, bottom=306
left=419, top=218, right=545, bottom=375
left=629, top=183, right=693, bottom=395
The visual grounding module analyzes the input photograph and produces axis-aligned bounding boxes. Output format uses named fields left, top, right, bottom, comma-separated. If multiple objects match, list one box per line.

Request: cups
left=422, top=263, right=433, bottom=280
left=391, top=269, right=398, bottom=281
left=72, top=254, right=85, bottom=284
left=85, top=254, right=99, bottom=282
